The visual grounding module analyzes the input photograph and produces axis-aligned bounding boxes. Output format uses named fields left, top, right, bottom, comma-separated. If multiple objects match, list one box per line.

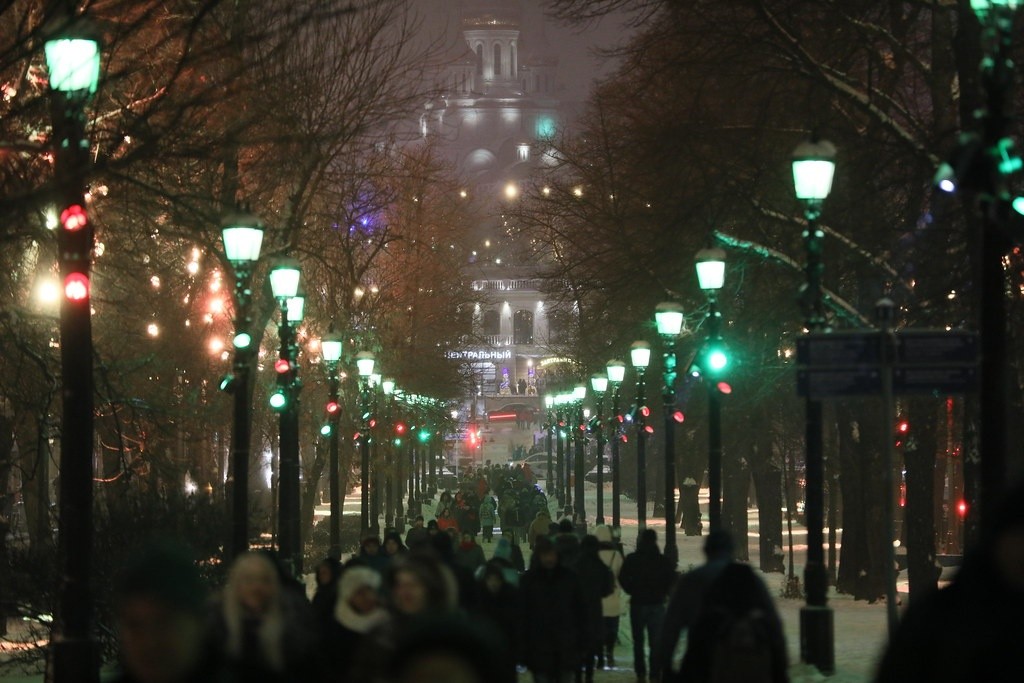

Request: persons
left=104, top=459, right=790, bottom=683
left=867, top=485, right=1024, bottom=683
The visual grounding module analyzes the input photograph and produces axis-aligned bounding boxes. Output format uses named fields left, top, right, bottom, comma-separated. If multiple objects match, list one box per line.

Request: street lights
left=393, top=388, right=406, bottom=534
left=655, top=295, right=684, bottom=569
left=405, top=392, right=445, bottom=520
left=563, top=390, right=573, bottom=514
left=606, top=358, right=626, bottom=530
left=355, top=346, right=375, bottom=537
left=368, top=370, right=382, bottom=536
left=790, top=137, right=837, bottom=675
left=320, top=323, right=344, bottom=545
left=546, top=393, right=566, bottom=508
left=222, top=198, right=263, bottom=556
left=45, top=13, right=103, bottom=683
left=630, top=335, right=652, bottom=532
left=268, top=260, right=307, bottom=577
left=382, top=378, right=396, bottom=529
left=592, top=369, right=608, bottom=526
left=693, top=236, right=728, bottom=529
left=574, top=379, right=586, bottom=520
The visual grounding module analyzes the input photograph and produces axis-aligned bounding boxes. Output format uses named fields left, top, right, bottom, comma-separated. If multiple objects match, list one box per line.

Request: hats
left=337, top=554, right=390, bottom=633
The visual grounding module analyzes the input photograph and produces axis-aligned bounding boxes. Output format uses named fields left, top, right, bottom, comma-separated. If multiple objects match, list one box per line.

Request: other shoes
left=596, top=659, right=604, bottom=668
left=585, top=668, right=594, bottom=683
left=607, top=655, right=616, bottom=667
left=574, top=668, right=583, bottom=683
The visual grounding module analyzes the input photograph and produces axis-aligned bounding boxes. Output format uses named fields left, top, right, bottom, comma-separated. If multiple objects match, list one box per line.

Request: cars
left=487, top=403, right=540, bottom=421
left=508, top=452, right=574, bottom=480
